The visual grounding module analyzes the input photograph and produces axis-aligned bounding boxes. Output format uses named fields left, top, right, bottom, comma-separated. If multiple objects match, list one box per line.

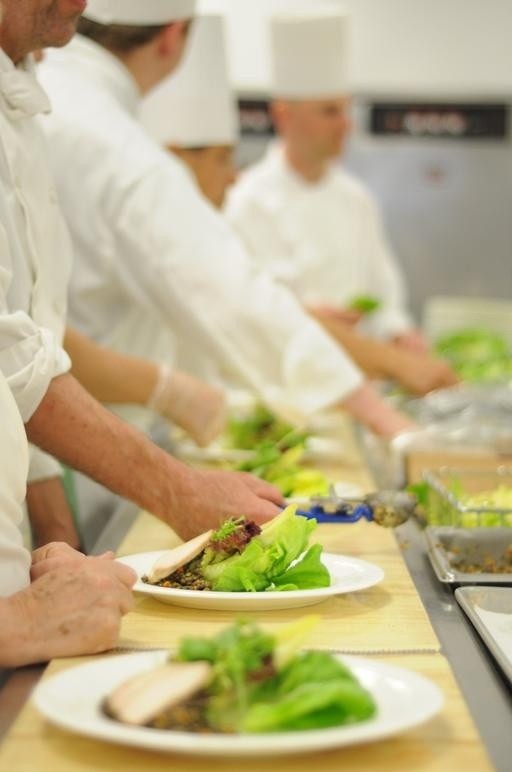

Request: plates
left=29, top=647, right=446, bottom=758
left=177, top=434, right=340, bottom=463
left=112, top=548, right=384, bottom=612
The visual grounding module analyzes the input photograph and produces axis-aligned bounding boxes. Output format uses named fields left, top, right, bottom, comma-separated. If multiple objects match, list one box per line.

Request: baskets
left=417, top=463, right=512, bottom=529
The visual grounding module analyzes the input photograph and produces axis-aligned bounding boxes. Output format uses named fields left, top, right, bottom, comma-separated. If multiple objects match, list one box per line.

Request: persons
left=36, top=20, right=418, bottom=450
left=163, top=145, right=459, bottom=393
left=60, top=323, right=237, bottom=447
left=0, top=371, right=142, bottom=669
left=222, top=96, right=430, bottom=353
left=0, top=0, right=291, bottom=554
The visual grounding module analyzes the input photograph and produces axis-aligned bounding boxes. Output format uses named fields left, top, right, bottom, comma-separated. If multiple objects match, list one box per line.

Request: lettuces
left=242, top=437, right=340, bottom=506
left=184, top=624, right=376, bottom=733
left=226, top=400, right=302, bottom=449
left=190, top=502, right=330, bottom=595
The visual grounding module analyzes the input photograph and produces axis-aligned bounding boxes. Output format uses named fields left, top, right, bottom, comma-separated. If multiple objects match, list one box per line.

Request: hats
left=82, top=0, right=198, bottom=28
left=138, top=14, right=240, bottom=148
left=269, top=14, right=360, bottom=101
